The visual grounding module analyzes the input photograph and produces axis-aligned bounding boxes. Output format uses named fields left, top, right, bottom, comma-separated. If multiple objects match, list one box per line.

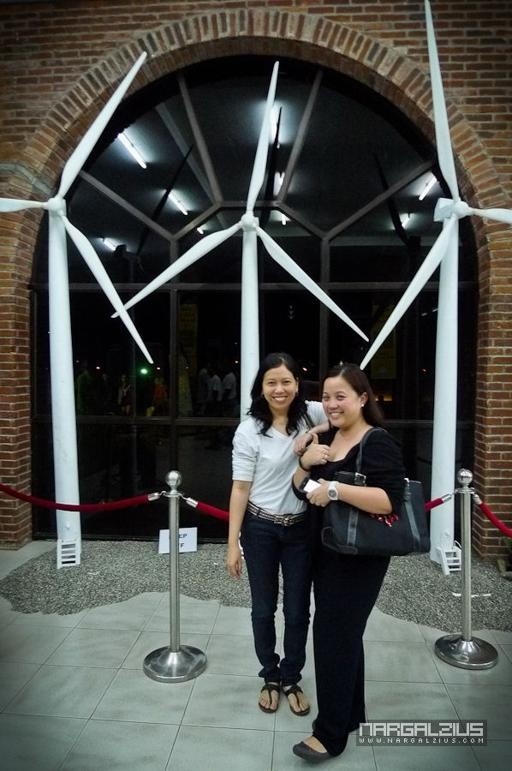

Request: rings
left=324, top=456, right=327, bottom=461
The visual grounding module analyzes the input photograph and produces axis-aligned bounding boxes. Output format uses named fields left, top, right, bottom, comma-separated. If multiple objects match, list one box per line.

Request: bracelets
left=298, top=456, right=311, bottom=474
left=305, top=431, right=312, bottom=435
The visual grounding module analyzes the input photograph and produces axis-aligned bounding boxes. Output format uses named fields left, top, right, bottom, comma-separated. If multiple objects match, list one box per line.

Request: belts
left=246, top=503, right=307, bottom=527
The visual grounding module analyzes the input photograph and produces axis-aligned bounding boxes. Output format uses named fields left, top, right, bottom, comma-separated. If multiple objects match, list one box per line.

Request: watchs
left=327, top=481, right=340, bottom=502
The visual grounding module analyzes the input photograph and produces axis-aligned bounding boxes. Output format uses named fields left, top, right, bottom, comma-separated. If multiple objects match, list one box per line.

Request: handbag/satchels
left=320, top=425, right=431, bottom=558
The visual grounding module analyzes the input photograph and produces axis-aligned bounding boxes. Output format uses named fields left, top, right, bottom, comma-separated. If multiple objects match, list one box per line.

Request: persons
left=225, top=350, right=337, bottom=716
left=74, top=356, right=237, bottom=423
left=289, top=363, right=407, bottom=767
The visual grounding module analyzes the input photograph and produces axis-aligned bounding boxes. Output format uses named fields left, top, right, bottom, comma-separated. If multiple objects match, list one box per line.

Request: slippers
left=282, top=683, right=310, bottom=716
left=258, top=683, right=280, bottom=713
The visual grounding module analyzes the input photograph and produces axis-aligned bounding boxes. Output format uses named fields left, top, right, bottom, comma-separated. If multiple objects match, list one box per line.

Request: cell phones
left=299, top=477, right=320, bottom=493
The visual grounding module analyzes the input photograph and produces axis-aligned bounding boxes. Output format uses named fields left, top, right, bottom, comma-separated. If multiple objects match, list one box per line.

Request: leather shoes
left=292, top=741, right=331, bottom=763
